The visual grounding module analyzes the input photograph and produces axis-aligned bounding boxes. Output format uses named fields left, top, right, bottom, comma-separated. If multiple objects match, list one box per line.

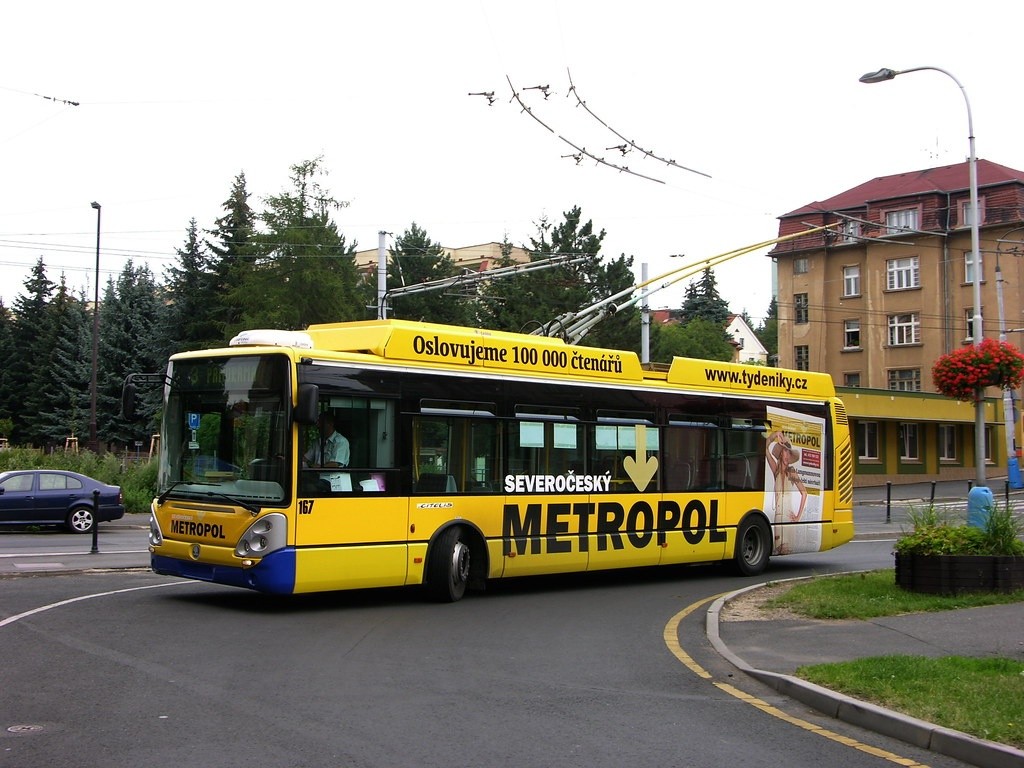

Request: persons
left=302, top=412, right=349, bottom=469
left=766, top=429, right=807, bottom=554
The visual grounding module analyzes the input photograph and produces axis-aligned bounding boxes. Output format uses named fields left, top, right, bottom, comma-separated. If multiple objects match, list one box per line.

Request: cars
left=1, top=469, right=126, bottom=534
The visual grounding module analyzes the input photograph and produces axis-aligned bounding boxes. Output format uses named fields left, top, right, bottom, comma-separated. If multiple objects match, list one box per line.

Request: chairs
left=415, top=451, right=752, bottom=491
left=338, top=421, right=365, bottom=482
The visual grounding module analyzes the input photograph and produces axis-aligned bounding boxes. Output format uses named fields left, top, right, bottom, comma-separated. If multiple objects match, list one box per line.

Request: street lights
left=857, top=65, right=988, bottom=486
left=88, top=201, right=101, bottom=442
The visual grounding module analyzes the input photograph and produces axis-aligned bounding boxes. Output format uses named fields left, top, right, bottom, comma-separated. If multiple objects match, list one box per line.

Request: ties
left=320, top=439, right=326, bottom=468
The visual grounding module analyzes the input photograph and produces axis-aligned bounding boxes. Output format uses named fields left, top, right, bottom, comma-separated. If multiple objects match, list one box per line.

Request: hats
left=771, top=436, right=799, bottom=463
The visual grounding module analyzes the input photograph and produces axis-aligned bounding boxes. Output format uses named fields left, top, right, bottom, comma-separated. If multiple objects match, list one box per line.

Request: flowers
left=930, top=338, right=1024, bottom=403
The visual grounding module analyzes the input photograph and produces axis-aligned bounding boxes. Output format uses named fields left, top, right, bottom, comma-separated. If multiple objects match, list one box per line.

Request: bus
left=118, top=317, right=855, bottom=604
